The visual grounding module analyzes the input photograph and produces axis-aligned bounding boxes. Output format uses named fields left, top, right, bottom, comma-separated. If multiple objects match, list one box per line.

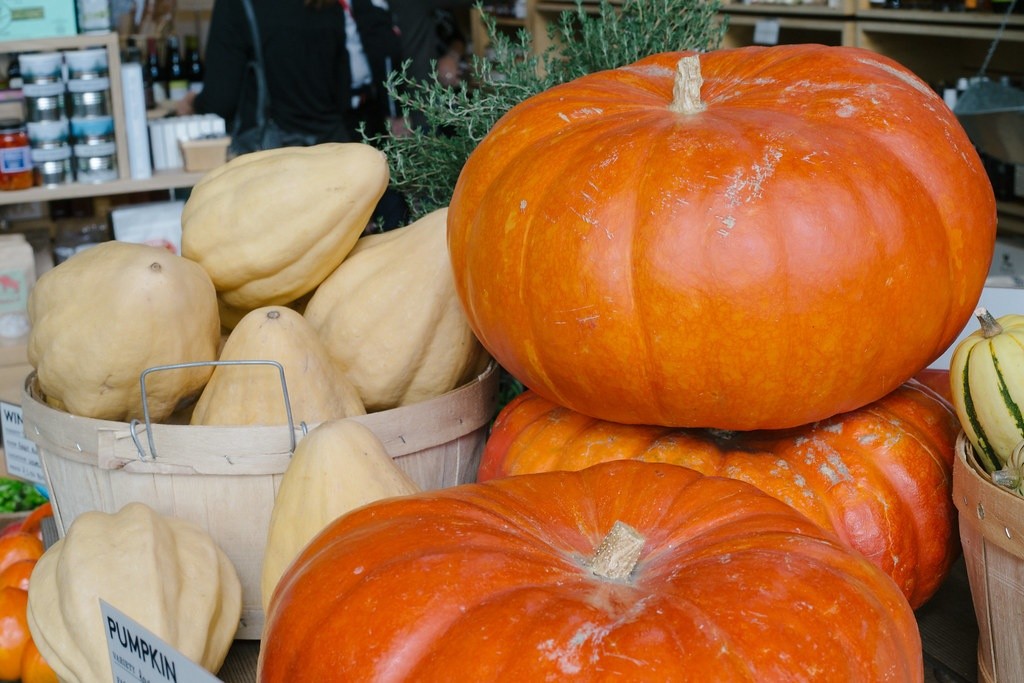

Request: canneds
left=0, top=126, right=35, bottom=191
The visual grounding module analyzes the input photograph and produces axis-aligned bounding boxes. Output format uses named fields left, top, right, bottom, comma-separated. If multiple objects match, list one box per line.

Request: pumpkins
left=27, top=140, right=491, bottom=429
left=0, top=501, right=242, bottom=683
left=248, top=43, right=1024, bottom=683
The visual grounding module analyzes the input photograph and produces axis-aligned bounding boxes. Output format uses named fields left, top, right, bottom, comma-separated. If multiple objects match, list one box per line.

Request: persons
left=175, top=0, right=471, bottom=239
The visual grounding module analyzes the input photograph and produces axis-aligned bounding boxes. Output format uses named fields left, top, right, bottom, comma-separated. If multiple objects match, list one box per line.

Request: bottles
left=120, top=34, right=204, bottom=111
left=0, top=118, right=34, bottom=190
left=0, top=234, right=36, bottom=339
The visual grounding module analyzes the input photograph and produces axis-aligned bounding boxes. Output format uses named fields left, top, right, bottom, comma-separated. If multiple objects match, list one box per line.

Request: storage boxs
left=0, top=233, right=34, bottom=315
left=111, top=201, right=183, bottom=255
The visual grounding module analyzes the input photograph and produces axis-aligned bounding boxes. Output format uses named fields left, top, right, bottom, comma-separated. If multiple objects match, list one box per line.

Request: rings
left=445, top=73, right=452, bottom=79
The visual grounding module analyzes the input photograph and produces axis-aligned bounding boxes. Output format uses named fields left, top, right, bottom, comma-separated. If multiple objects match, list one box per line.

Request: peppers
left=0, top=476, right=51, bottom=513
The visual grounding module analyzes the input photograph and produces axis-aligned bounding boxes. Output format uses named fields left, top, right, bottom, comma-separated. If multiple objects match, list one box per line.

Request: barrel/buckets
left=951, top=429, right=1023, bottom=683
left=20, top=360, right=496, bottom=643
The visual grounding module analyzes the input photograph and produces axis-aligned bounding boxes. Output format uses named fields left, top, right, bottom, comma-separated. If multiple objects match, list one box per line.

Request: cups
left=17, top=47, right=119, bottom=190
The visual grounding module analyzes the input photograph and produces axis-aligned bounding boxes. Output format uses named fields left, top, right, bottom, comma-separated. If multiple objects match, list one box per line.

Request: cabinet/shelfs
left=0, top=33, right=238, bottom=417
